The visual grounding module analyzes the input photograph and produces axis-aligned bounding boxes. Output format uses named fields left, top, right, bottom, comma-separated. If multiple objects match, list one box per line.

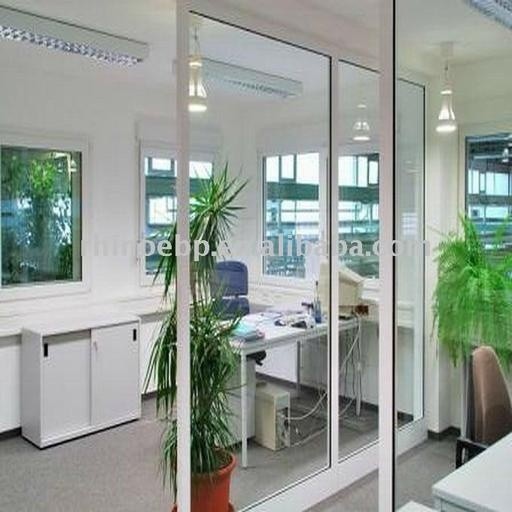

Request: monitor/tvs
left=318, top=260, right=364, bottom=320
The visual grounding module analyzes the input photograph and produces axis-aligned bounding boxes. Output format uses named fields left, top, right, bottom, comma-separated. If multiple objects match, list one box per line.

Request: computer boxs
left=253, top=385, right=291, bottom=452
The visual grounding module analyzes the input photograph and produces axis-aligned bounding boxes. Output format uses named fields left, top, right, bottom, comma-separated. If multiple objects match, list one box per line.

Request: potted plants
left=139, top=157, right=254, bottom=512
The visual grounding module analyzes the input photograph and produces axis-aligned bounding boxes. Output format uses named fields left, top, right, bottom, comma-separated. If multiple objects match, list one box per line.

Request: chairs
left=454, top=344, right=512, bottom=470
left=208, top=260, right=249, bottom=319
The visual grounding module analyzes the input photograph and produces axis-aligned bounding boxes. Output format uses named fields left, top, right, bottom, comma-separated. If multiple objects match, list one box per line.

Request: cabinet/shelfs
left=21, top=311, right=142, bottom=449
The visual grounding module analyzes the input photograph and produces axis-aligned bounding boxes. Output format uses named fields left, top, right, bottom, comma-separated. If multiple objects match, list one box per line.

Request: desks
left=431, top=430, right=512, bottom=512
left=210, top=299, right=375, bottom=469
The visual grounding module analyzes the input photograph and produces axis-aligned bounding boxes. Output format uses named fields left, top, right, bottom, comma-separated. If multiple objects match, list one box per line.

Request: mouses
left=275, top=319, right=286, bottom=326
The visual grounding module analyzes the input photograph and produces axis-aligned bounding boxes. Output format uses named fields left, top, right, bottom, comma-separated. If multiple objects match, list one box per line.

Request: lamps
left=351, top=94, right=372, bottom=143
left=187, top=21, right=209, bottom=113
left=434, top=59, right=460, bottom=133
